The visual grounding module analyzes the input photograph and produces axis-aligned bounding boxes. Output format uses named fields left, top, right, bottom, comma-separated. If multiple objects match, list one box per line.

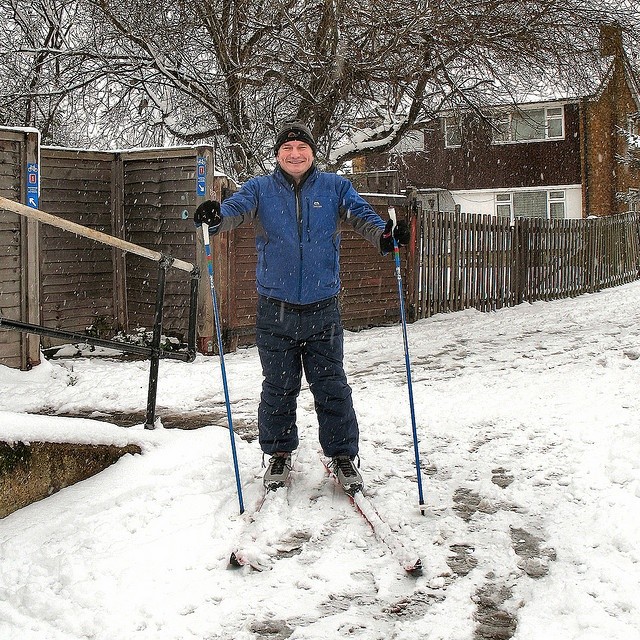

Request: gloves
left=194, top=200, right=220, bottom=233
left=383, top=220, right=410, bottom=254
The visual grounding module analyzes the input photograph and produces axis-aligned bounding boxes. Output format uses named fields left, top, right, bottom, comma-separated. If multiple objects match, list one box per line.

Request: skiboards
left=230, top=445, right=422, bottom=571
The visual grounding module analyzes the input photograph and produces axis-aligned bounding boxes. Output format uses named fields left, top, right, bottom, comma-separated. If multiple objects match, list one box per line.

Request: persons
left=193, top=122, right=410, bottom=494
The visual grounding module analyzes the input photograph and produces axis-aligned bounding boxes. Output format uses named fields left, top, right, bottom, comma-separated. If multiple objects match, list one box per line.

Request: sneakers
left=263, top=452, right=291, bottom=490
left=327, top=454, right=364, bottom=493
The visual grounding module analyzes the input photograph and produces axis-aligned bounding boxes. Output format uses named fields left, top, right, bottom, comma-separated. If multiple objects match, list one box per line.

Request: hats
left=275, top=123, right=316, bottom=157
